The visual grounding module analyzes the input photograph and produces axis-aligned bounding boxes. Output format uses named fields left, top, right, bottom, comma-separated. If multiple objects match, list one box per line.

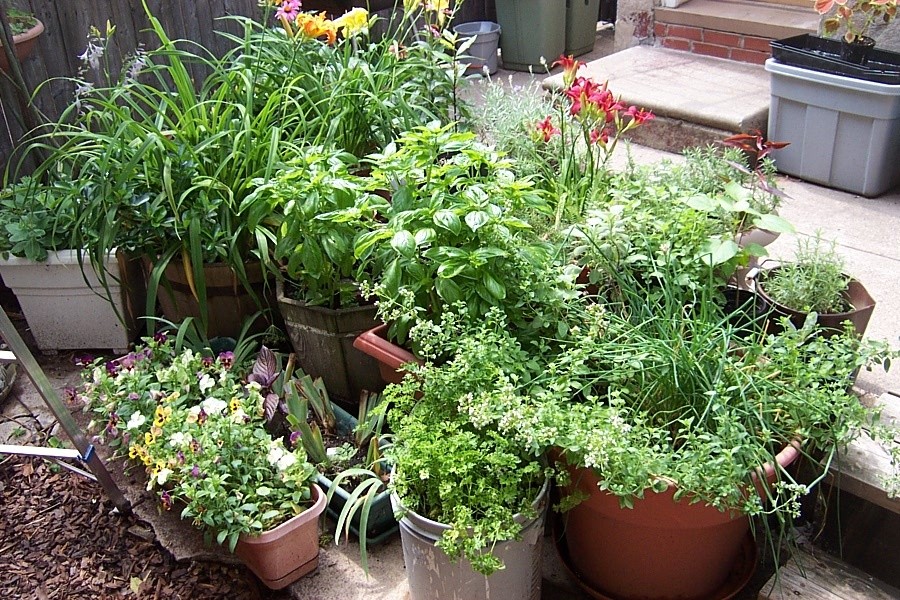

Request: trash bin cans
left=495, top=0, right=568, bottom=73
left=563, top=0, right=600, bottom=58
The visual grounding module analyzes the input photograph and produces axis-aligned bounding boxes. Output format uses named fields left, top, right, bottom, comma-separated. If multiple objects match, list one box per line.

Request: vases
left=839, top=34, right=876, bottom=66
left=229, top=479, right=328, bottom=588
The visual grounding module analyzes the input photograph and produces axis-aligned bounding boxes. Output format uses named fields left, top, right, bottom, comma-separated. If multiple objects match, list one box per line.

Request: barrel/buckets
left=453, top=21, right=500, bottom=75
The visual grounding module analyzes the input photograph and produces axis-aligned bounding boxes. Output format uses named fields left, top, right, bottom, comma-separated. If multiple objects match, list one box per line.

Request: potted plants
left=1, top=0, right=874, bottom=600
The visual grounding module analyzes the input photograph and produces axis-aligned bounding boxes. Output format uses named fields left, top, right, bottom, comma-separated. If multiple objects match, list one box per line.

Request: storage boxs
left=764, top=33, right=900, bottom=196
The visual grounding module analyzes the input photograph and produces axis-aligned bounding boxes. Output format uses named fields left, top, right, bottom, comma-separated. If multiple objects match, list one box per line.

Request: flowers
left=815, top=0, right=900, bottom=43
left=71, top=325, right=312, bottom=548
left=527, top=54, right=655, bottom=222
left=240, top=0, right=459, bottom=149
